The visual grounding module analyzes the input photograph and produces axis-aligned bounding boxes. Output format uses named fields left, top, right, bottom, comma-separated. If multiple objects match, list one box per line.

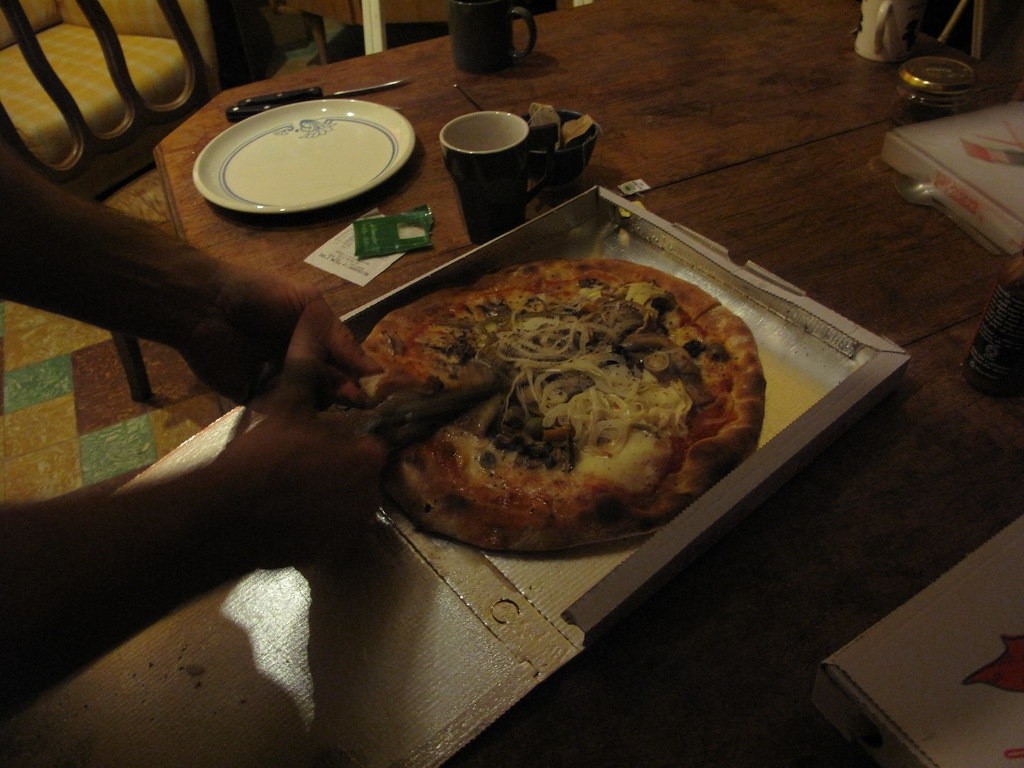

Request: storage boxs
left=0, top=182, right=915, bottom=768
left=882, top=101, right=1024, bottom=256
left=810, top=516, right=1024, bottom=768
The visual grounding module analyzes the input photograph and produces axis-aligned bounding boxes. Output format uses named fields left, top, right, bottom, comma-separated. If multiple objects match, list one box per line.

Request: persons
left=0, top=151, right=384, bottom=723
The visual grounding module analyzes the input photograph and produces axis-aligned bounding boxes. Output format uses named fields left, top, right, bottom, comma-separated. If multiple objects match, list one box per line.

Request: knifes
left=224, top=75, right=415, bottom=119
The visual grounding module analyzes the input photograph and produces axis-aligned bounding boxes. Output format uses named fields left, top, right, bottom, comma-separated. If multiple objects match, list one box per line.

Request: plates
left=192, top=98, right=416, bottom=212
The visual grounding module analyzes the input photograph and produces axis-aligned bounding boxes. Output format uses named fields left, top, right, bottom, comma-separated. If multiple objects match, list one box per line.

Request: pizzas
left=352, top=259, right=768, bottom=552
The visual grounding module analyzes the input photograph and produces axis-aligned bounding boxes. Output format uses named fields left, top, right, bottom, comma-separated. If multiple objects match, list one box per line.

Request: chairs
left=0, top=0, right=221, bottom=206
left=0, top=0, right=219, bottom=404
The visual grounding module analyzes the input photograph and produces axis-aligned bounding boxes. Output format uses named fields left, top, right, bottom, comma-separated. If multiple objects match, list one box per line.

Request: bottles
left=965, top=248, right=1024, bottom=399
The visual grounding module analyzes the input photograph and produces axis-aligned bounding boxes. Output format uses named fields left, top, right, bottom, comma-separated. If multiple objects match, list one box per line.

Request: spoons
left=894, top=173, right=1002, bottom=256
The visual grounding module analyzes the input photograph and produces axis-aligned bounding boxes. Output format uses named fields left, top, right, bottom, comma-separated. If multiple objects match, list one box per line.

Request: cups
left=447, top=0, right=538, bottom=72
left=439, top=111, right=555, bottom=244
left=855, top=0, right=927, bottom=63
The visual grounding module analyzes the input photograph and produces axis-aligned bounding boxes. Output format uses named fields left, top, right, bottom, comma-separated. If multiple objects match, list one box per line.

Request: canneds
left=886, top=57, right=976, bottom=130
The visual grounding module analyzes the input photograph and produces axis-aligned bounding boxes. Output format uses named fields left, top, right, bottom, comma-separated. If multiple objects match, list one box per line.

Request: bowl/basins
left=522, top=109, right=600, bottom=188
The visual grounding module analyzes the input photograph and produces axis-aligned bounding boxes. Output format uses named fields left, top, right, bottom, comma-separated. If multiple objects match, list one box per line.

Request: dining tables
left=154, top=0, right=1023, bottom=768
